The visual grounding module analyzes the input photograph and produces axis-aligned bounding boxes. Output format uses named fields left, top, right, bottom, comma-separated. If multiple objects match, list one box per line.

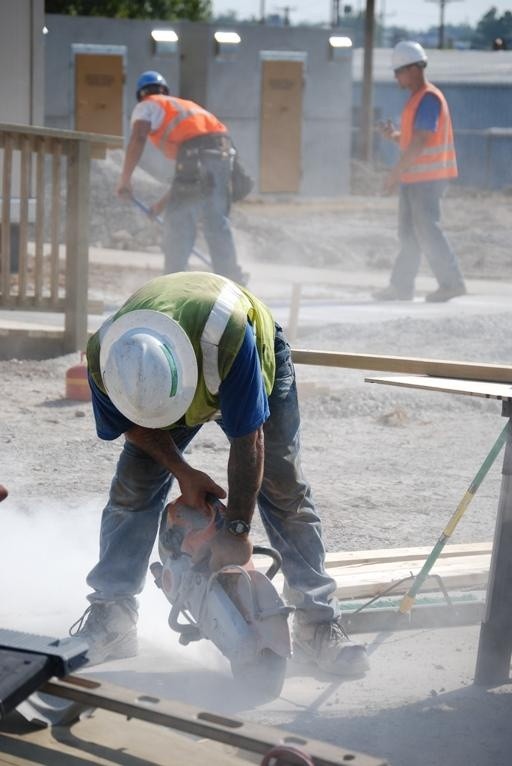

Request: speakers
left=59, top=598, right=139, bottom=673
left=290, top=611, right=371, bottom=676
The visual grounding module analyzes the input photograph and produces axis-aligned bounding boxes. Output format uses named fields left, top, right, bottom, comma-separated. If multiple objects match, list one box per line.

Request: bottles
left=99, top=309, right=198, bottom=430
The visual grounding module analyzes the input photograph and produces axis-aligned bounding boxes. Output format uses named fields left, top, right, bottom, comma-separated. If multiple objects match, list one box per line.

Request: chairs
left=371, top=282, right=415, bottom=301
left=425, top=278, right=466, bottom=303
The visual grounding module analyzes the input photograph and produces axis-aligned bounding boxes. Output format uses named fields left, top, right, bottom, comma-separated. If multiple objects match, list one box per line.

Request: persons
left=376, top=38, right=470, bottom=302
left=115, top=69, right=253, bottom=292
left=60, top=270, right=379, bottom=680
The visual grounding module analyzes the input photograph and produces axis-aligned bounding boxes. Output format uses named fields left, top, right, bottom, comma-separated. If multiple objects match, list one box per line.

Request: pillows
left=135, top=70, right=170, bottom=100
left=386, top=40, right=428, bottom=71
